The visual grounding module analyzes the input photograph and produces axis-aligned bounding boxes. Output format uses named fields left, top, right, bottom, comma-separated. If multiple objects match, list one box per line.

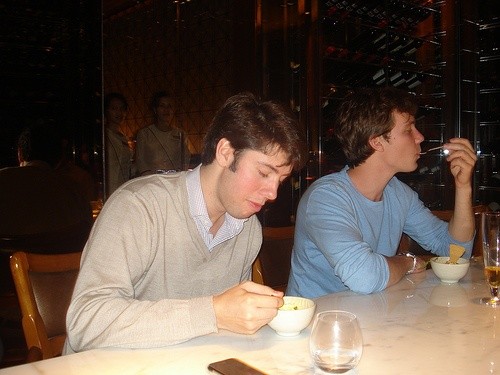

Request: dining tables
left=0, top=258, right=500, bottom=374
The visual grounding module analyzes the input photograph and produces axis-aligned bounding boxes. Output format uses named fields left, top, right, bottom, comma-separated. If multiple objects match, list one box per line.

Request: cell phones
left=208, top=357, right=268, bottom=375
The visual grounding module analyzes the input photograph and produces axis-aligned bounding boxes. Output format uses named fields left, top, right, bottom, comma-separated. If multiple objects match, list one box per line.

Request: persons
left=0, top=89, right=193, bottom=253
left=61, top=91, right=310, bottom=356
left=284, top=83, right=479, bottom=299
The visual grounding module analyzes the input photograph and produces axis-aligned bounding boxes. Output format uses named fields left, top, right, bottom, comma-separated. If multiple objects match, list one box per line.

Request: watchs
left=397, top=252, right=417, bottom=275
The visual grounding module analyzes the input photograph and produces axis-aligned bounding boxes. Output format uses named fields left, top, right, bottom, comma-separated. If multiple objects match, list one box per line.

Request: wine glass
left=480, top=211, right=500, bottom=308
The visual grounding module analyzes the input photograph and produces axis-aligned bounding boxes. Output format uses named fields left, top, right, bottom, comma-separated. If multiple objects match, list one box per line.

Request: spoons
left=419, top=145, right=443, bottom=154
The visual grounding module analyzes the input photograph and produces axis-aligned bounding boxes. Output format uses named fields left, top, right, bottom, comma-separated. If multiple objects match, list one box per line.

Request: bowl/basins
left=430, top=256, right=470, bottom=283
left=267, top=296, right=315, bottom=337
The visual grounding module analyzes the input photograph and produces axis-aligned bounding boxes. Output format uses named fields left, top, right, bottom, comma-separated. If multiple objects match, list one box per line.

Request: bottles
left=477, top=141, right=480, bottom=153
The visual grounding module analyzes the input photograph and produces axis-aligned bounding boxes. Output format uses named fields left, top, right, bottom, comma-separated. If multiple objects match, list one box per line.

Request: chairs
left=0, top=217, right=89, bottom=337
left=396, top=204, right=490, bottom=276
left=252, top=227, right=295, bottom=296
left=9, top=252, right=82, bottom=363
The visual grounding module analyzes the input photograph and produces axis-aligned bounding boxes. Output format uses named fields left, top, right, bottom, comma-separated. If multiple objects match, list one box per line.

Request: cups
left=309, top=310, right=364, bottom=375
